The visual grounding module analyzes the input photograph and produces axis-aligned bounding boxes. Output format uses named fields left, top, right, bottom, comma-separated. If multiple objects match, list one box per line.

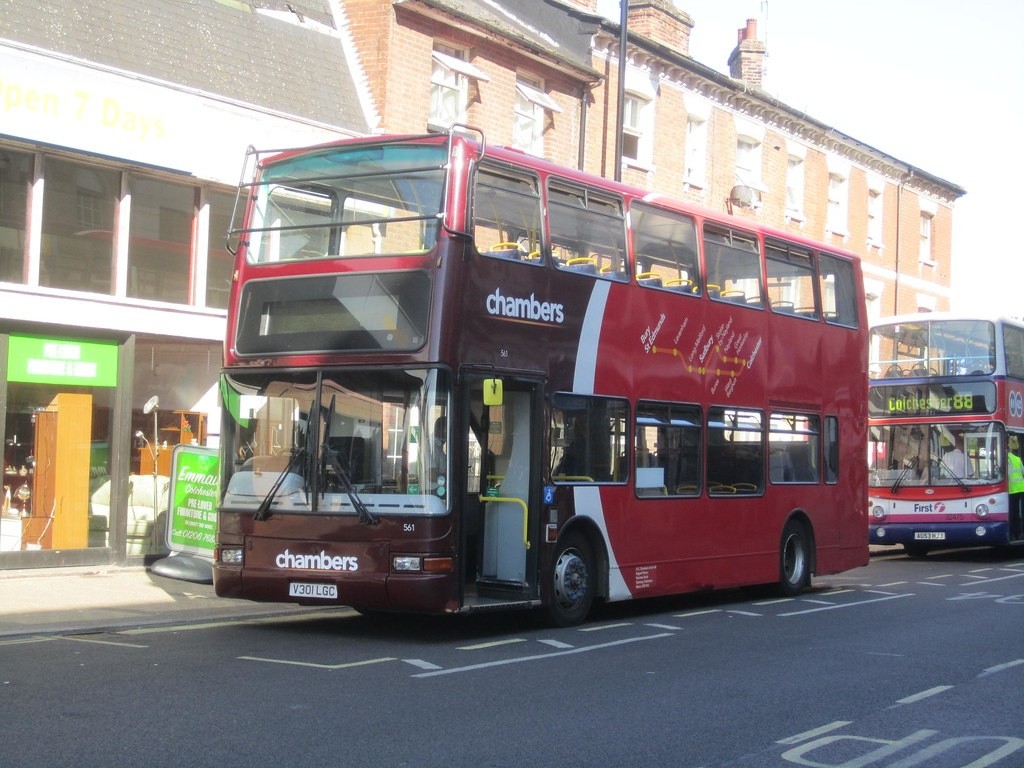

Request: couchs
left=88, top=474, right=172, bottom=552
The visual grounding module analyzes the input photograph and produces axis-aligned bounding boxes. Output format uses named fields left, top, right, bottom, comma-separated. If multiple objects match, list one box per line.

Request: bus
left=867, top=313, right=1024, bottom=557
left=211, top=121, right=873, bottom=628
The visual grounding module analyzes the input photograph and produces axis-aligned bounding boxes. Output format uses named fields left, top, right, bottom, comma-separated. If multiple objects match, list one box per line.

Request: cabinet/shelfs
left=0, top=386, right=94, bottom=552
left=91, top=407, right=208, bottom=446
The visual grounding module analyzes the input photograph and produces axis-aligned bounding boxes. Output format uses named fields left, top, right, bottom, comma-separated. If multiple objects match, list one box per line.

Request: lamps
left=134, top=395, right=160, bottom=554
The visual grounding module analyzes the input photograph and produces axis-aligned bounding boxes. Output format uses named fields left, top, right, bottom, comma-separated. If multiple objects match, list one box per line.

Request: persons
left=1007, top=450, right=1024, bottom=538
left=940, top=431, right=975, bottom=479
left=417, top=416, right=447, bottom=501
